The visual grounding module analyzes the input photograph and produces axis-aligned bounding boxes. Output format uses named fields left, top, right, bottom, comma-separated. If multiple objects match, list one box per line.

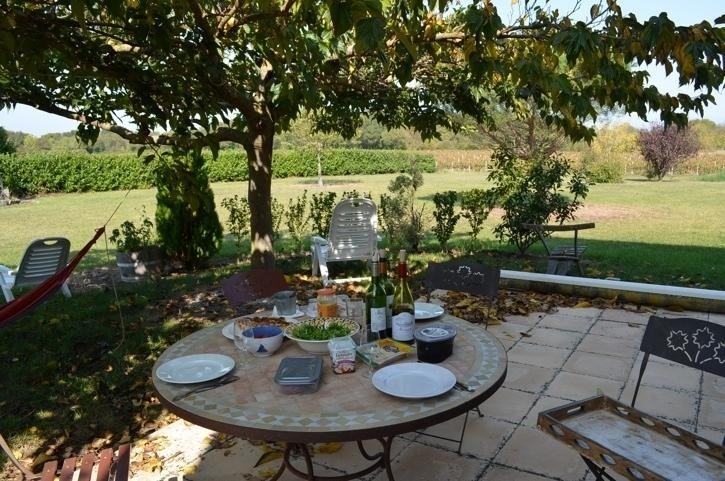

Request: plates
left=372, top=364, right=457, bottom=401
left=414, top=300, right=445, bottom=321
left=220, top=313, right=296, bottom=342
left=155, top=353, right=236, bottom=386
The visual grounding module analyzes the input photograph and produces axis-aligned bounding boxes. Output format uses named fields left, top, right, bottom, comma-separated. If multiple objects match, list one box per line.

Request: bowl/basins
left=243, top=327, right=283, bottom=357
left=284, top=318, right=360, bottom=355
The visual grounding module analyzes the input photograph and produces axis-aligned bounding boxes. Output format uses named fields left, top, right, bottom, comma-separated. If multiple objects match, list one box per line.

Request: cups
left=302, top=287, right=363, bottom=323
left=272, top=291, right=295, bottom=318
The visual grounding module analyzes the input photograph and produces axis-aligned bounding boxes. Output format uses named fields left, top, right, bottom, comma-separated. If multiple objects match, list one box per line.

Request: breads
left=236, top=316, right=291, bottom=332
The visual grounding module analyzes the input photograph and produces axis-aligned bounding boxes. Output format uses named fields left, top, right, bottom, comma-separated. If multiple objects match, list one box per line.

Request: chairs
left=580, top=315, right=725, bottom=481
left=222, top=266, right=303, bottom=318
left=0, top=237, right=72, bottom=304
left=0, top=431, right=130, bottom=481
left=413, top=254, right=500, bottom=456
left=522, top=222, right=595, bottom=276
left=310, top=198, right=383, bottom=288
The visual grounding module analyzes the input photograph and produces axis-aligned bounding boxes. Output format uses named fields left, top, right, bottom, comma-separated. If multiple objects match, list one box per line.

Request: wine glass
left=359, top=324, right=380, bottom=379
left=234, top=320, right=255, bottom=364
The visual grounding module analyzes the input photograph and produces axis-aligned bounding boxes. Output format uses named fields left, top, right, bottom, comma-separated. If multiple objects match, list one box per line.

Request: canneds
left=317, top=288, right=337, bottom=317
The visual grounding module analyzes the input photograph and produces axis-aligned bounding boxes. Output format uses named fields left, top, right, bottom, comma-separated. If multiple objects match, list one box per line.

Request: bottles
left=364, top=248, right=417, bottom=344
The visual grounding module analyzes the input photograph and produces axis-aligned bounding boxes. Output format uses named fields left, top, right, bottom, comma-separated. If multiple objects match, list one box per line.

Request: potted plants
left=108, top=204, right=161, bottom=282
